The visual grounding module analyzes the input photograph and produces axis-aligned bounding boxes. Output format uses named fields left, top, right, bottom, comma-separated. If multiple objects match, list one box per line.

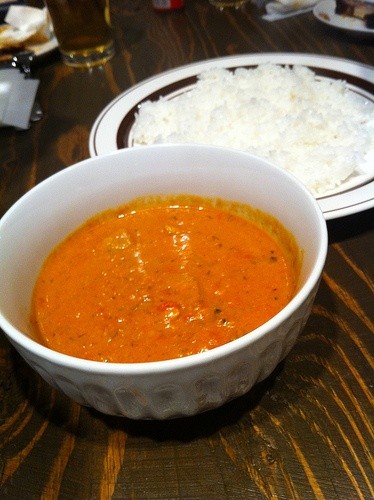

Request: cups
left=45, top=0, right=116, bottom=67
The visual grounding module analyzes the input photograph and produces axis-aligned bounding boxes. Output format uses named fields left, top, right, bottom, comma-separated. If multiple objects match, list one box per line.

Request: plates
left=0, top=23, right=59, bottom=61
left=89, top=52, right=374, bottom=220
left=313, top=0, right=374, bottom=34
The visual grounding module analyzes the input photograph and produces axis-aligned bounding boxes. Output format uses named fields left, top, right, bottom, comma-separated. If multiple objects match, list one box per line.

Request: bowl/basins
left=0, top=143, right=328, bottom=420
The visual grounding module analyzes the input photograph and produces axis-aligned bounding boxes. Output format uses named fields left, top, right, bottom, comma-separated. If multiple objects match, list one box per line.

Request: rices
left=131, top=62, right=374, bottom=196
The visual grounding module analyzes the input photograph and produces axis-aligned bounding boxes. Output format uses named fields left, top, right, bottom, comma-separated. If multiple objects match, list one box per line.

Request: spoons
left=13, top=50, right=43, bottom=122
